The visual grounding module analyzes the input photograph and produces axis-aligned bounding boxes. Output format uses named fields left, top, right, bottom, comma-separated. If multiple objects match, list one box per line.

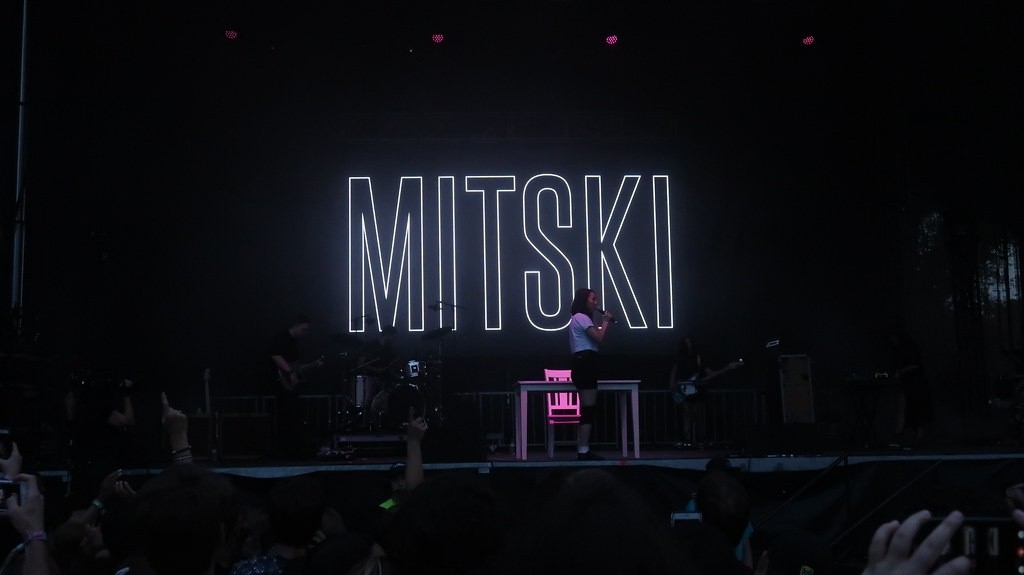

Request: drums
left=371, top=382, right=428, bottom=430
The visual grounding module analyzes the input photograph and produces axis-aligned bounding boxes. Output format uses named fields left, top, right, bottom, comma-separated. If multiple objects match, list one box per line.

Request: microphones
left=428, top=304, right=441, bottom=311
left=596, top=306, right=617, bottom=324
left=365, top=317, right=376, bottom=325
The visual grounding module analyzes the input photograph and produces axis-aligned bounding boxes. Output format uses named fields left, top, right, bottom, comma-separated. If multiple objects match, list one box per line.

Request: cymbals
left=421, top=326, right=453, bottom=341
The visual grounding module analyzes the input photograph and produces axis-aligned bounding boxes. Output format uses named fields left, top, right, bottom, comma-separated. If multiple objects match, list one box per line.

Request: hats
left=384, top=462, right=406, bottom=479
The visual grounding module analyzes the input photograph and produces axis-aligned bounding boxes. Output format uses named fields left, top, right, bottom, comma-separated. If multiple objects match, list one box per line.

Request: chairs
left=544, top=368, right=582, bottom=458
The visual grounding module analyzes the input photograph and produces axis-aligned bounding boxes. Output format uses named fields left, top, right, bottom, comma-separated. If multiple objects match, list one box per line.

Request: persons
left=889, top=329, right=933, bottom=447
left=0, top=393, right=1024, bottom=575
left=365, top=325, right=398, bottom=416
left=269, top=316, right=312, bottom=393
left=671, top=336, right=718, bottom=448
left=82, top=378, right=135, bottom=473
left=568, top=288, right=614, bottom=460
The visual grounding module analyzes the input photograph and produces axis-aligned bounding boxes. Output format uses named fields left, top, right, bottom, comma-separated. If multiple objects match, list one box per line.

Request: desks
left=512, top=380, right=642, bottom=461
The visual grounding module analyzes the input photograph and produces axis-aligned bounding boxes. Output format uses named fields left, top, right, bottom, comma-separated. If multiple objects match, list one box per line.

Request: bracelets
left=92, top=500, right=103, bottom=510
left=175, top=456, right=193, bottom=462
left=170, top=446, right=192, bottom=456
left=24, top=531, right=48, bottom=542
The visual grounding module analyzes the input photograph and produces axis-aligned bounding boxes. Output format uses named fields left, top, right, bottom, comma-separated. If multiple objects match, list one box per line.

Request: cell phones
left=670, top=513, right=702, bottom=528
left=0, top=480, right=29, bottom=512
left=910, top=518, right=1024, bottom=575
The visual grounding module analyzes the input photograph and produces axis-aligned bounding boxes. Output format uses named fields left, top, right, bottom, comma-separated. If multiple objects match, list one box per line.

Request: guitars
left=276, top=357, right=325, bottom=393
left=675, top=359, right=745, bottom=398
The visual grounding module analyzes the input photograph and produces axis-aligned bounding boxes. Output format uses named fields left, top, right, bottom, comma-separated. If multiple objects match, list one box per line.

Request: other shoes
left=570, top=447, right=603, bottom=460
